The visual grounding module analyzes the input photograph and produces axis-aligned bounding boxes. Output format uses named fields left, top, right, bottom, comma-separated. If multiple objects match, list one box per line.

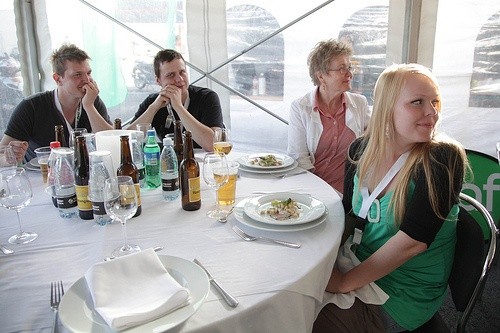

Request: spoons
left=217, top=206, right=234, bottom=223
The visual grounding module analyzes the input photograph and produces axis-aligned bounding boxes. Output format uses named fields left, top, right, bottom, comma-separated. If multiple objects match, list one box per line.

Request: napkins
left=85, top=247, right=192, bottom=331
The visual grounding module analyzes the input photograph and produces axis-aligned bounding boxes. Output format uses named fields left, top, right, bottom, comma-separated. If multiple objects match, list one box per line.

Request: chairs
left=396, top=192, right=496, bottom=333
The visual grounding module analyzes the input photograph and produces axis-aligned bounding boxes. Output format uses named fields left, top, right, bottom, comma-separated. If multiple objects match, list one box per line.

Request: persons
left=286, top=38, right=371, bottom=193
left=312, top=63, right=475, bottom=333
left=0, top=42, right=114, bottom=167
left=124, top=49, right=227, bottom=151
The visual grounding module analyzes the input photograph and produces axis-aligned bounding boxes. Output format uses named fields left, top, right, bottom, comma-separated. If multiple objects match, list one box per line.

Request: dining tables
left=0, top=149, right=390, bottom=333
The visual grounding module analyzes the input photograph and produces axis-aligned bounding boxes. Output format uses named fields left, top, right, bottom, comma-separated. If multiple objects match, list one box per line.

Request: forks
left=232, top=226, right=301, bottom=248
left=49, top=280, right=65, bottom=333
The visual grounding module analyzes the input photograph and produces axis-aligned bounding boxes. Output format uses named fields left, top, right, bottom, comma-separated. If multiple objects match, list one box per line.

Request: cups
left=68, top=128, right=88, bottom=149
left=129, top=133, right=146, bottom=170
left=165, top=133, right=185, bottom=148
left=88, top=150, right=121, bottom=203
left=136, top=123, right=152, bottom=144
left=34, top=146, right=52, bottom=184
left=214, top=163, right=239, bottom=207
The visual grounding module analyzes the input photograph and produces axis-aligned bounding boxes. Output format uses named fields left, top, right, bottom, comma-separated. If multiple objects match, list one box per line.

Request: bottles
left=142, top=116, right=202, bottom=211
left=116, top=135, right=142, bottom=218
left=44, top=119, right=121, bottom=226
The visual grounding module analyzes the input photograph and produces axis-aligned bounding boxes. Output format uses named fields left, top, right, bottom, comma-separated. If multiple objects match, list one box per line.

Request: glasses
left=329, top=66, right=354, bottom=75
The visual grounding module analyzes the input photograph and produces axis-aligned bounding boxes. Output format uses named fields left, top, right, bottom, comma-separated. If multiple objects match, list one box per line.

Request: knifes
left=192, top=259, right=239, bottom=307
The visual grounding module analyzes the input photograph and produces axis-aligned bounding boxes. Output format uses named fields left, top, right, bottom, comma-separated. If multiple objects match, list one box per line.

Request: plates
left=234, top=156, right=298, bottom=175
left=58, top=254, right=210, bottom=333
left=244, top=192, right=326, bottom=225
left=23, top=157, right=41, bottom=172
left=239, top=152, right=294, bottom=168
left=233, top=197, right=329, bottom=232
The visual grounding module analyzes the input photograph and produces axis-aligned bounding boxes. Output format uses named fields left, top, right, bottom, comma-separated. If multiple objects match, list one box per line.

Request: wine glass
left=0, top=145, right=39, bottom=244
left=203, top=127, right=233, bottom=219
left=104, top=175, right=141, bottom=258
